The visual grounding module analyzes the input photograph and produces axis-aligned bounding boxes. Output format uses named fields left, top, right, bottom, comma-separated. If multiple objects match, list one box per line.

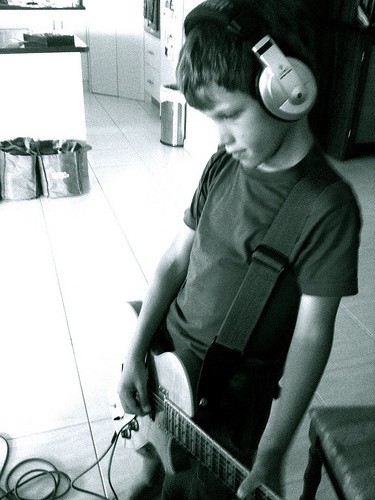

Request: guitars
left=110, top=299, right=289, bottom=500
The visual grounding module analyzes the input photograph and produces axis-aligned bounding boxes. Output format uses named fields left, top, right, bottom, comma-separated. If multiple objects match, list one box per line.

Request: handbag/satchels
left=38, top=138, right=91, bottom=199
left=0, top=136, right=41, bottom=202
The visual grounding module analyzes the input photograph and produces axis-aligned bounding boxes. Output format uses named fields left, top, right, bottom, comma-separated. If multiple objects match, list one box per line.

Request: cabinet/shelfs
left=143, top=30, right=160, bottom=103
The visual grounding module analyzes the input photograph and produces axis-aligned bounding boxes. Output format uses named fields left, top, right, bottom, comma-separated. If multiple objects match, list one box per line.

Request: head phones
left=185, top=5, right=318, bottom=121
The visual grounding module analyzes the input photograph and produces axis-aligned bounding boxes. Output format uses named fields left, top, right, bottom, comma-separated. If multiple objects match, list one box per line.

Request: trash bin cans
left=158, top=84, right=188, bottom=148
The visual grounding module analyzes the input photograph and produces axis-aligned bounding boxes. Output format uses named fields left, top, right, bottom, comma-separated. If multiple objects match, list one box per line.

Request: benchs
left=297, top=405, right=375, bottom=500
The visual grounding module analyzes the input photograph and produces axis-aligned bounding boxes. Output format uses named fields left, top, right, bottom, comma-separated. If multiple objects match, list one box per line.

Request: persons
left=115, top=0, right=365, bottom=500
